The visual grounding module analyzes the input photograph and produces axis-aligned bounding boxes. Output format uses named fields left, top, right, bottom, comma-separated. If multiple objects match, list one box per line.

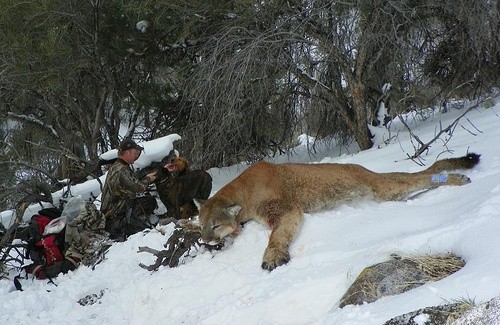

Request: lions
left=192, top=152, right=482, bottom=272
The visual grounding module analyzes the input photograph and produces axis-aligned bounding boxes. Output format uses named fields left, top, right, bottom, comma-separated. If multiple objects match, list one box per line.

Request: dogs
left=139, top=156, right=212, bottom=223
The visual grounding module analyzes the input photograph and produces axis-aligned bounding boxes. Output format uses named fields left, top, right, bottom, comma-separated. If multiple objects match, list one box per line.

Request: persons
left=102, top=137, right=178, bottom=241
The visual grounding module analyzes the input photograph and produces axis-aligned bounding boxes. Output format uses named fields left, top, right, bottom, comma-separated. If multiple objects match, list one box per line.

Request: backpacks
left=64, top=202, right=108, bottom=266
left=26, top=208, right=81, bottom=279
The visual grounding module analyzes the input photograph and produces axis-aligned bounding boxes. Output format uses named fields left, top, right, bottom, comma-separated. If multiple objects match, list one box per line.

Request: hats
left=119, top=140, right=144, bottom=150
